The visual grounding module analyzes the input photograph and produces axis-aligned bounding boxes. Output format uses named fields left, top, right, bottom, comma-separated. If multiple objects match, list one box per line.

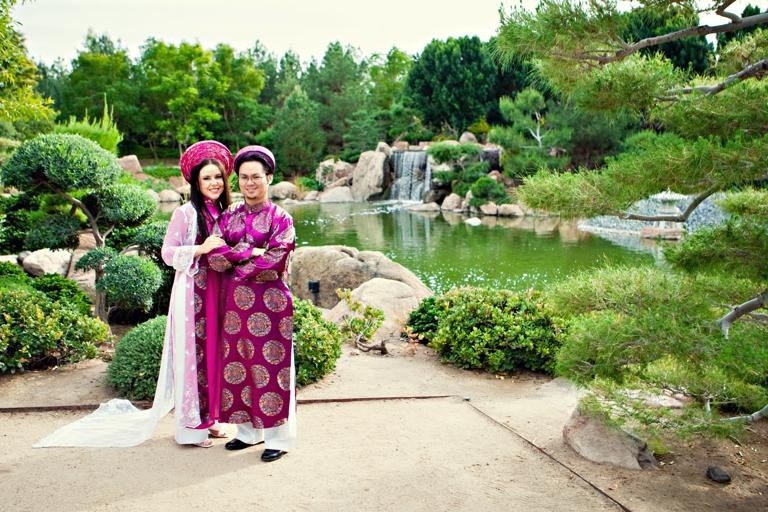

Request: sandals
left=192, top=438, right=214, bottom=447
left=207, top=426, right=228, bottom=438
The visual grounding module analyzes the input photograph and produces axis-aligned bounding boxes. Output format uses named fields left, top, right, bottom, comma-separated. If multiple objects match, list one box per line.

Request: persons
left=206, top=144, right=297, bottom=462
left=159, top=140, right=234, bottom=448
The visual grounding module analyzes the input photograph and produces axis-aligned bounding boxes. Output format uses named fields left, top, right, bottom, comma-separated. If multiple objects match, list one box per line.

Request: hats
left=233, top=145, right=275, bottom=175
left=180, top=140, right=234, bottom=184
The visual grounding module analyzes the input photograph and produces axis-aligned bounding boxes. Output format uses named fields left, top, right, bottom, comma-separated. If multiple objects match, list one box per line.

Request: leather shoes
left=262, top=449, right=287, bottom=462
left=225, top=438, right=264, bottom=450
